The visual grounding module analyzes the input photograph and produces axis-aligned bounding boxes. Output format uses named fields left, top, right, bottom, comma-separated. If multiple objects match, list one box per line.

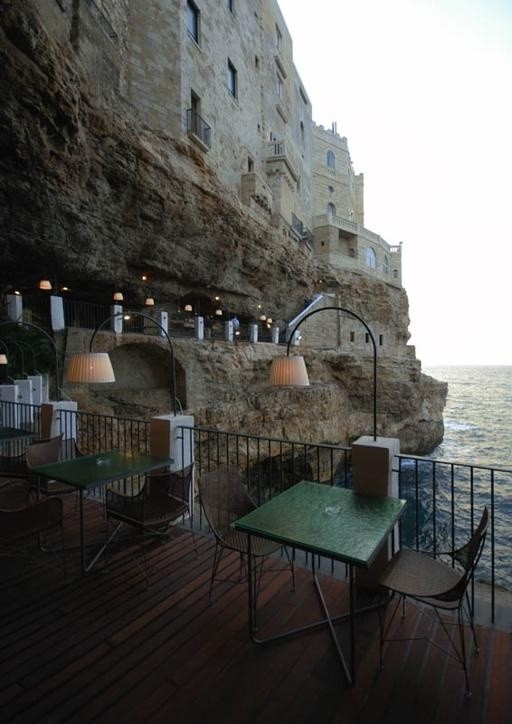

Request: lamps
left=0, top=280, right=272, bottom=417
left=270, top=307, right=377, bottom=441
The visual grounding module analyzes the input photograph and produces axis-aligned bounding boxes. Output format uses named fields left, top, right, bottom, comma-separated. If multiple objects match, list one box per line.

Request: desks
left=0, top=426, right=37, bottom=440
left=235, top=481, right=407, bottom=687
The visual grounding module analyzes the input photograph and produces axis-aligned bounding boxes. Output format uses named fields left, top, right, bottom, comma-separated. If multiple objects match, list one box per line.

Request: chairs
left=378, top=506, right=489, bottom=698
left=0, top=432, right=199, bottom=586
left=197, top=466, right=295, bottom=618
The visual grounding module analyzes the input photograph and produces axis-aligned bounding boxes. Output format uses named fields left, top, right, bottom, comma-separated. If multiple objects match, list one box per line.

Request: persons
left=204, top=315, right=213, bottom=338
left=232, top=315, right=240, bottom=339
left=304, top=294, right=310, bottom=308
